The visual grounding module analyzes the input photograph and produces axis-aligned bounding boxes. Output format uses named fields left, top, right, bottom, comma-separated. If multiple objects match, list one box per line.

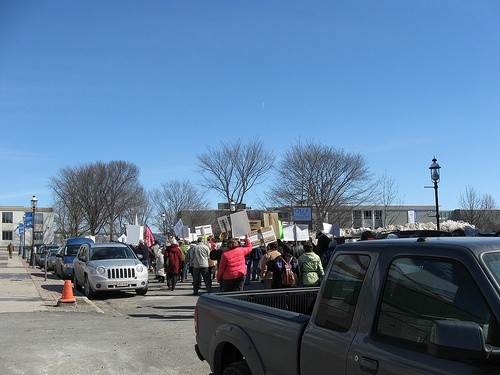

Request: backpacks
left=278, top=255, right=295, bottom=285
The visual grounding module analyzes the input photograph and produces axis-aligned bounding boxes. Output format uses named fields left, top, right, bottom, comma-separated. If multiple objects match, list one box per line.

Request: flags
left=146, top=226, right=154, bottom=246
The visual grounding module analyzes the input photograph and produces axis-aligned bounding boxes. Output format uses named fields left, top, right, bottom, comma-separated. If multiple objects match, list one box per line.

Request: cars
left=36, top=244, right=60, bottom=271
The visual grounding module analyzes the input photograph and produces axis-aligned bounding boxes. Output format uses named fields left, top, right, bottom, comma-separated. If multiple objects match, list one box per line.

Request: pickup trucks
left=192, top=235, right=500, bottom=375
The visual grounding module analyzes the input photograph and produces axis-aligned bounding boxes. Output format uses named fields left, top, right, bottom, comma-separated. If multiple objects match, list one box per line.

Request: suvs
left=53, top=237, right=95, bottom=280
left=72, top=242, right=150, bottom=299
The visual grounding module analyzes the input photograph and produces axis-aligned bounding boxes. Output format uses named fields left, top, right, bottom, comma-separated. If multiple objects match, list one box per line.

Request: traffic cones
left=59, top=279, right=77, bottom=303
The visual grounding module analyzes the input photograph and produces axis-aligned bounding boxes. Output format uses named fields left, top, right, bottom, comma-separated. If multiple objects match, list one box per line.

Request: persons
left=245, top=240, right=307, bottom=289
left=298, top=240, right=325, bottom=287
left=217, top=234, right=252, bottom=292
left=452, top=229, right=465, bottom=237
left=204, top=232, right=230, bottom=291
left=177, top=237, right=198, bottom=282
left=130, top=240, right=167, bottom=283
left=385, top=233, right=398, bottom=239
left=7, top=242, right=15, bottom=258
left=164, top=236, right=182, bottom=291
left=360, top=231, right=376, bottom=240
left=191, top=237, right=210, bottom=295
left=308, top=231, right=339, bottom=270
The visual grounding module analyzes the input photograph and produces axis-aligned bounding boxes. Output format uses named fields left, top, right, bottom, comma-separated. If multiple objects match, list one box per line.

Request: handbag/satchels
left=164, top=248, right=170, bottom=267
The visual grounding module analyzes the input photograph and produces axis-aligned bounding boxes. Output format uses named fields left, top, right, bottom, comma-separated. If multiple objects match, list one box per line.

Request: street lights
left=29, top=195, right=39, bottom=269
left=162, top=212, right=166, bottom=236
left=429, top=154, right=441, bottom=231
left=20, top=213, right=26, bottom=260
